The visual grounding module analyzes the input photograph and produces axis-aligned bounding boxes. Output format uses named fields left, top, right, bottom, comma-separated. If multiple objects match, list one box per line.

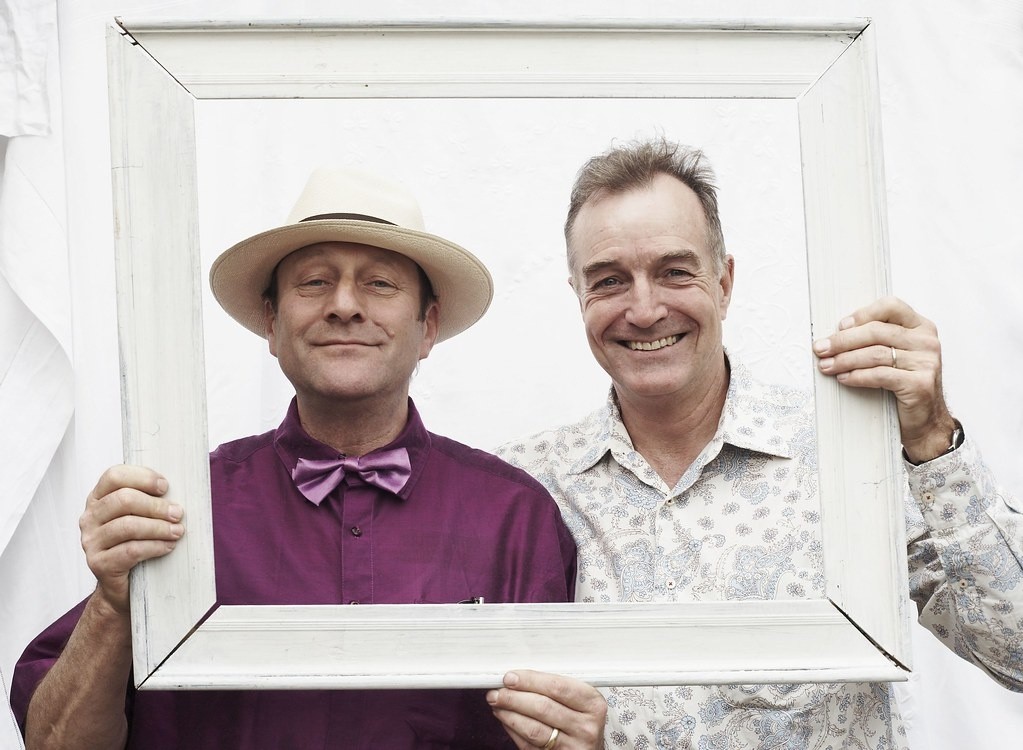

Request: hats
left=208, top=163, right=493, bottom=345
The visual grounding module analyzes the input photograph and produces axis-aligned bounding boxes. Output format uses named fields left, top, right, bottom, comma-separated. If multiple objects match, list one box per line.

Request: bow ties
left=291, top=446, right=413, bottom=507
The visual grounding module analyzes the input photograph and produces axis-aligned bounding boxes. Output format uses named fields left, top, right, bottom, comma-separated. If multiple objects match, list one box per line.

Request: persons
left=487, top=127, right=1023, bottom=750
left=8, top=175, right=611, bottom=750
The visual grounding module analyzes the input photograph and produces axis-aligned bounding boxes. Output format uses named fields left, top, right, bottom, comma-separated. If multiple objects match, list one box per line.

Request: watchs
left=939, top=423, right=965, bottom=456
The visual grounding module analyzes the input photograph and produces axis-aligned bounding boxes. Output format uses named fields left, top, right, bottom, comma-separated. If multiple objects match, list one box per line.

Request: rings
left=890, top=347, right=896, bottom=369
left=539, top=727, right=560, bottom=750
